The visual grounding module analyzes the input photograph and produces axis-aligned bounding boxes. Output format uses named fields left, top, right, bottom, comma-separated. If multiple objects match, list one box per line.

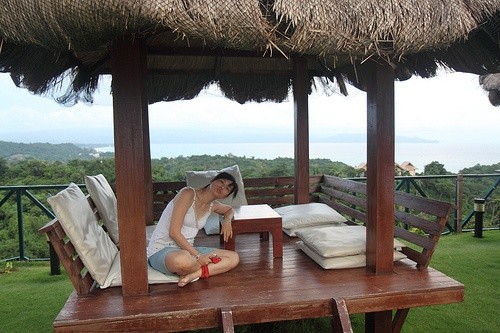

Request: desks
left=216, top=203, right=284, bottom=258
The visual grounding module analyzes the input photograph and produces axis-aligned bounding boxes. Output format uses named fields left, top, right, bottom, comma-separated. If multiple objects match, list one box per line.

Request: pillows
left=98, top=251, right=200, bottom=289
left=273, top=200, right=409, bottom=272
left=47, top=182, right=119, bottom=290
left=146, top=224, right=157, bottom=244
left=185, top=164, right=248, bottom=235
left=84, top=173, right=118, bottom=244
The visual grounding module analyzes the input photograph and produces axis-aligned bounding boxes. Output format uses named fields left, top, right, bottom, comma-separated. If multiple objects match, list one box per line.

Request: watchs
left=195, top=253, right=201, bottom=260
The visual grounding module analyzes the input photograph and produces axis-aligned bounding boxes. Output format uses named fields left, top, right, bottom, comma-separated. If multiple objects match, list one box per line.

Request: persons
left=146, top=173, right=240, bottom=287
